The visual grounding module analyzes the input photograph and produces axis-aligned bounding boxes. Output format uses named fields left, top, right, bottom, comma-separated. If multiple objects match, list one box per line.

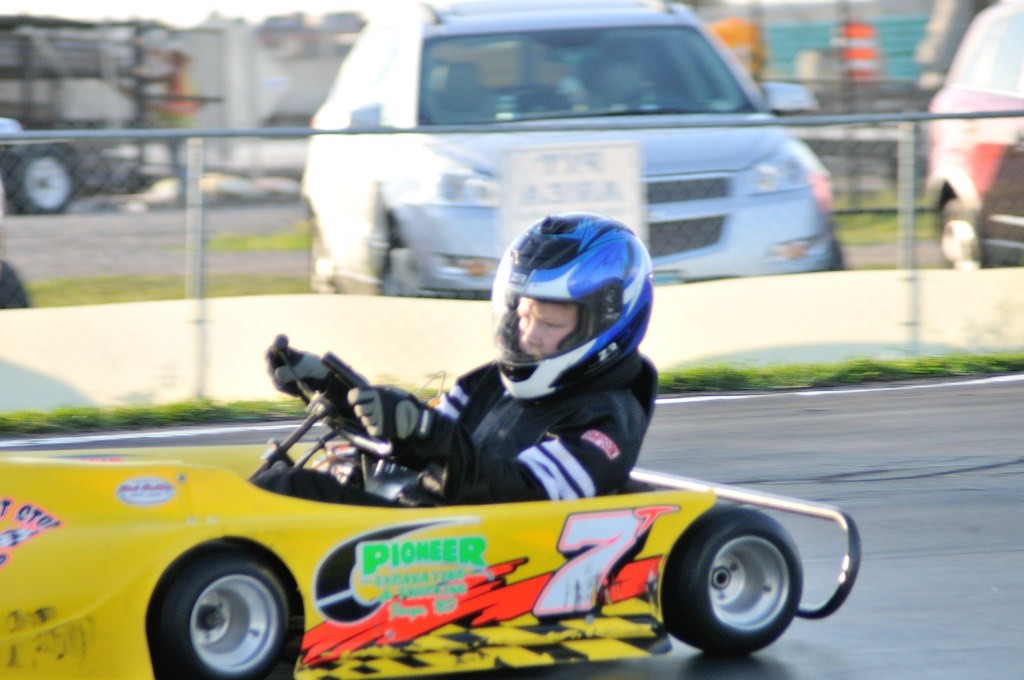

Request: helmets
left=493, top=216, right=652, bottom=401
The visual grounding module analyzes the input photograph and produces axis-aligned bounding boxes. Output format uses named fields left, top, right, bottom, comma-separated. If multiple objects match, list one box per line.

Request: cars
left=302, top=0, right=847, bottom=302
left=922, top=1, right=1024, bottom=268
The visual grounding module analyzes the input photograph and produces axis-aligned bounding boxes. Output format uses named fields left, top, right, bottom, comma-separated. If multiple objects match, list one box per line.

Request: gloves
left=266, top=333, right=339, bottom=403
left=348, top=387, right=451, bottom=457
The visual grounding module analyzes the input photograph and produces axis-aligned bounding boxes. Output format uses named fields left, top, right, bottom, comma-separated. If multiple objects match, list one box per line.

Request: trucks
left=0, top=9, right=221, bottom=219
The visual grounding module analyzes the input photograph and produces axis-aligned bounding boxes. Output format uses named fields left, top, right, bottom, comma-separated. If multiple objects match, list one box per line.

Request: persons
left=266, top=211, right=659, bottom=508
